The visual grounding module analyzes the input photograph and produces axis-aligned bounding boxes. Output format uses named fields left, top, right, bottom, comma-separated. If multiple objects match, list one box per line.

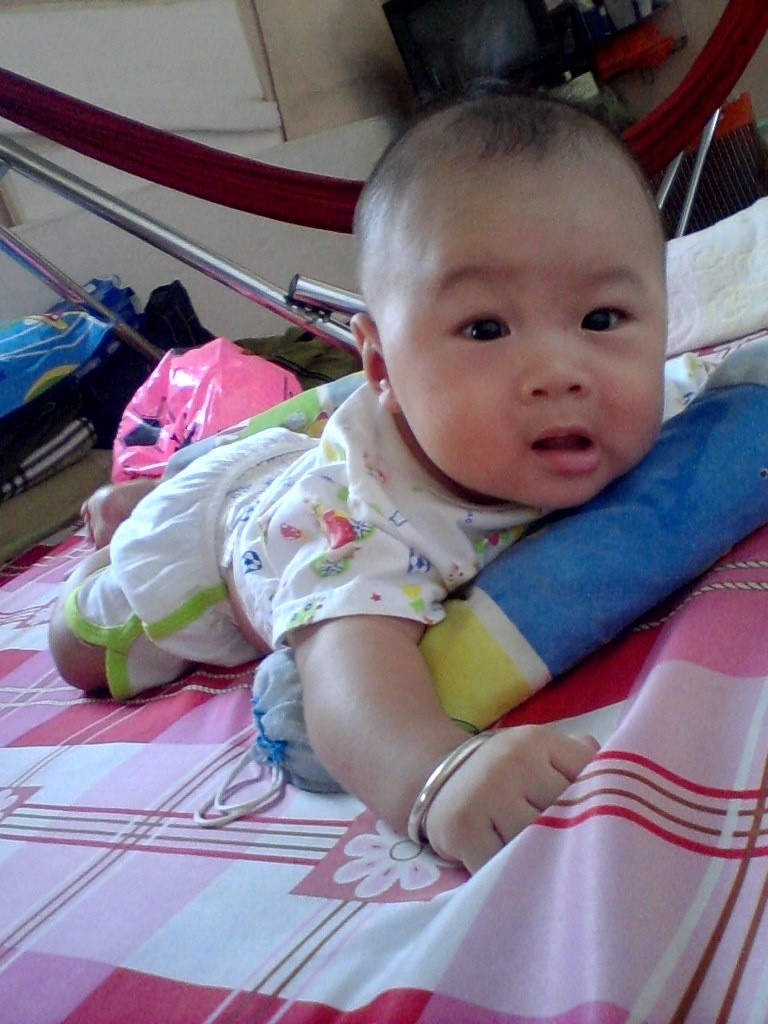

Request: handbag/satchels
left=111, top=340, right=305, bottom=479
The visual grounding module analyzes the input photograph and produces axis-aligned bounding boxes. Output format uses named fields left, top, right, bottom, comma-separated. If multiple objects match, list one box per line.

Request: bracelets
left=407, top=728, right=491, bottom=849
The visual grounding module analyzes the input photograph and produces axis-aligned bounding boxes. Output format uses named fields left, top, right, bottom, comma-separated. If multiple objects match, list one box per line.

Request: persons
left=49, top=94, right=667, bottom=877
